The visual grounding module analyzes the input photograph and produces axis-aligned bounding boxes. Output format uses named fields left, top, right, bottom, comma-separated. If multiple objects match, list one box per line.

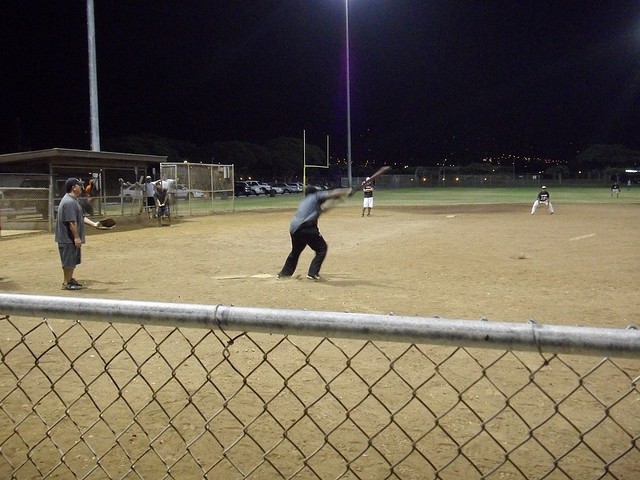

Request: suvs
left=11, top=179, right=65, bottom=213
left=226, top=183, right=250, bottom=197
left=168, top=184, right=204, bottom=201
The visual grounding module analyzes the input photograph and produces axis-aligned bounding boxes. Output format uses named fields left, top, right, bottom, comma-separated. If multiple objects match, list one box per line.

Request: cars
left=120, top=184, right=156, bottom=203
left=258, top=183, right=271, bottom=195
left=246, top=182, right=263, bottom=196
left=290, top=183, right=300, bottom=194
left=273, top=183, right=294, bottom=194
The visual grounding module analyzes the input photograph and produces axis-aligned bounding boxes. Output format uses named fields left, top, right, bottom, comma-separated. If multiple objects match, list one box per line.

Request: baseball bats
left=348, top=166, right=391, bottom=197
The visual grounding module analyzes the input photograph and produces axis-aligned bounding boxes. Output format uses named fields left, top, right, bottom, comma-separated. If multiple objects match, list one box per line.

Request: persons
left=136, top=176, right=161, bottom=216
left=54, top=178, right=115, bottom=288
left=610, top=183, right=621, bottom=198
left=531, top=186, right=554, bottom=215
left=361, top=176, right=374, bottom=216
left=277, top=186, right=353, bottom=281
left=153, top=182, right=175, bottom=228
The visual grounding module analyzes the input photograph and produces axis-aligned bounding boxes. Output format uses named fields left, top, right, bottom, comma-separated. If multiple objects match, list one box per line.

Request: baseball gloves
left=96, top=217, right=117, bottom=230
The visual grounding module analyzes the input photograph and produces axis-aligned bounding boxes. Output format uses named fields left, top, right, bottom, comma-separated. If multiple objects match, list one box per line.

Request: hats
left=64, top=178, right=85, bottom=187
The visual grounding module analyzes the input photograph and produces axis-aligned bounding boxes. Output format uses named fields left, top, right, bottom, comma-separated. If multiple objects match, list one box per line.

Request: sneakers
left=276, top=273, right=293, bottom=281
left=61, top=278, right=82, bottom=290
left=306, top=274, right=320, bottom=280
left=541, top=185, right=547, bottom=189
